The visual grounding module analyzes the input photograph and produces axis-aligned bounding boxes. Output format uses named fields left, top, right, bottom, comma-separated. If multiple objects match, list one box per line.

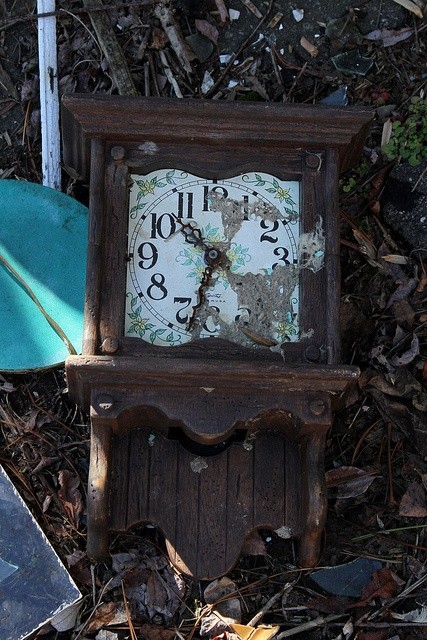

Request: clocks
left=59, top=89, right=376, bottom=584
